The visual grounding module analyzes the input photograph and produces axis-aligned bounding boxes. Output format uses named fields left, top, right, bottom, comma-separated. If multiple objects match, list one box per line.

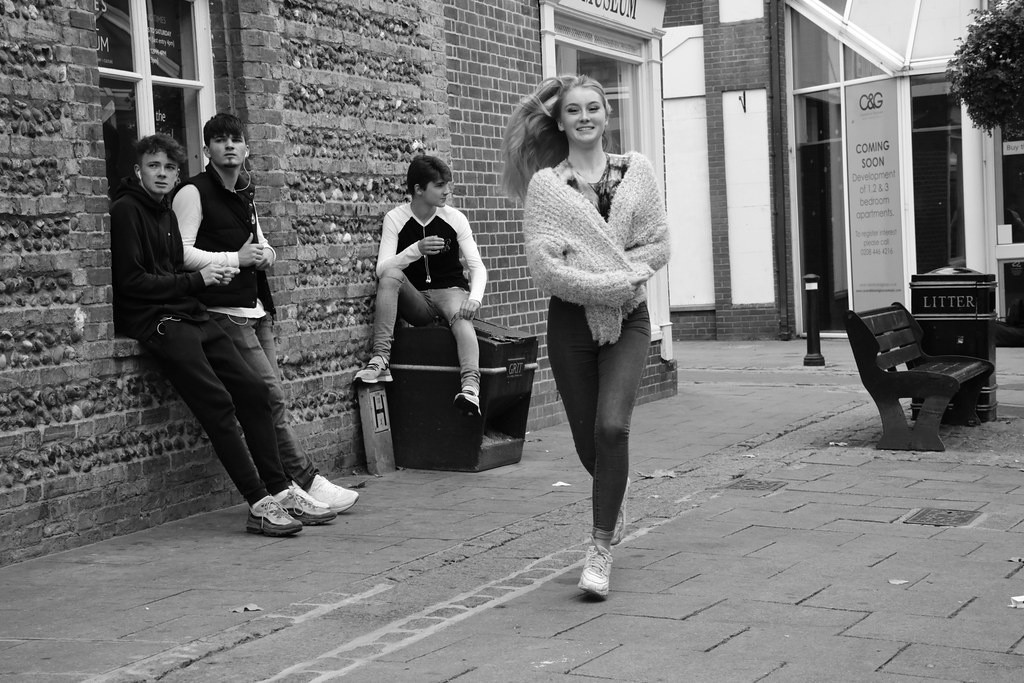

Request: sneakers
left=578, top=475, right=629, bottom=598
left=454, top=384, right=482, bottom=418
left=352, top=355, right=393, bottom=384
left=310, top=475, right=360, bottom=513
left=247, top=494, right=338, bottom=535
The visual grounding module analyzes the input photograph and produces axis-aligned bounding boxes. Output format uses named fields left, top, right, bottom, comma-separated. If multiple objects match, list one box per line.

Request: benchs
left=843, top=301, right=994, bottom=453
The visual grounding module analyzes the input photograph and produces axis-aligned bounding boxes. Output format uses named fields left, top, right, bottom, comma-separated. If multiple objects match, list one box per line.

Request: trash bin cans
left=909, top=266, right=997, bottom=423
left=384, top=317, right=541, bottom=472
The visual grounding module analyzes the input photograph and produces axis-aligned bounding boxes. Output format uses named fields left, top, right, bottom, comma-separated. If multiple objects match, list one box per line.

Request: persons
left=170, top=112, right=359, bottom=516
left=351, top=155, right=488, bottom=417
left=504, top=75, right=670, bottom=601
left=112, top=132, right=337, bottom=536
